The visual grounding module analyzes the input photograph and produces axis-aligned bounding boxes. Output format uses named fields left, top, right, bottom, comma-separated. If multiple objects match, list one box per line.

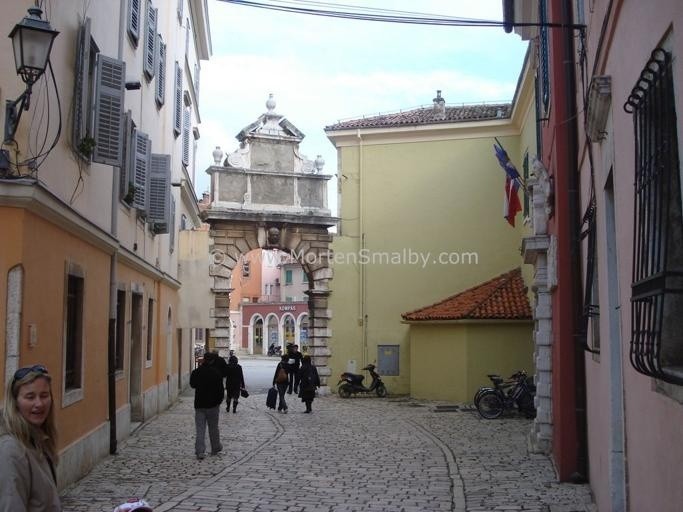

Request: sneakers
left=278, top=408, right=312, bottom=414
left=211, top=443, right=222, bottom=455
left=196, top=445, right=207, bottom=460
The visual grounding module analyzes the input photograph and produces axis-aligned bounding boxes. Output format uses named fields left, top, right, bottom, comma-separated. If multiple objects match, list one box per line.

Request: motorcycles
left=336, top=358, right=387, bottom=398
left=266, top=342, right=283, bottom=357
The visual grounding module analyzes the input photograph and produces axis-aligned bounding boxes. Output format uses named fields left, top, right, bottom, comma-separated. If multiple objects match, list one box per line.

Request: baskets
left=525, top=376, right=535, bottom=391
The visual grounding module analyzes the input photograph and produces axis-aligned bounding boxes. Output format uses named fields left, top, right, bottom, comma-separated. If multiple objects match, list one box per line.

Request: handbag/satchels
left=241, top=389, right=249, bottom=398
left=276, top=363, right=287, bottom=384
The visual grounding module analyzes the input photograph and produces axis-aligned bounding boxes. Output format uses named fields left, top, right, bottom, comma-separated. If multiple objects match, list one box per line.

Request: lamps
left=4, top=6, right=59, bottom=144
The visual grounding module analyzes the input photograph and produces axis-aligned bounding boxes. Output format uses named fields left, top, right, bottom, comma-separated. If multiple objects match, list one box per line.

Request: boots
left=226, top=401, right=230, bottom=412
left=233, top=401, right=237, bottom=413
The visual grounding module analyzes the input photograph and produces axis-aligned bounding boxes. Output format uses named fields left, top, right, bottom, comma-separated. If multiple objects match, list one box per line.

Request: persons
left=229, top=350, right=235, bottom=357
left=189, top=352, right=222, bottom=458
left=299, top=345, right=308, bottom=359
left=293, top=355, right=319, bottom=413
left=0, top=363, right=64, bottom=512
left=210, top=348, right=227, bottom=381
left=285, top=345, right=303, bottom=395
left=272, top=354, right=295, bottom=413
left=223, top=356, right=248, bottom=412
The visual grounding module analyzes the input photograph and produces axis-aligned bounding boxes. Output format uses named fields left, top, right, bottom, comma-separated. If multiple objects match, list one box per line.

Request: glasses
left=12, top=365, right=49, bottom=388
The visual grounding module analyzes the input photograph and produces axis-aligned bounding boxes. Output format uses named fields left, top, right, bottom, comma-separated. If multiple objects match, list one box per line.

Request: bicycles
left=473, top=368, right=536, bottom=420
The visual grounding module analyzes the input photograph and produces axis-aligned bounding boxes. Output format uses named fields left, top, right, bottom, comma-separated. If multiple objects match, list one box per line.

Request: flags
left=503, top=172, right=519, bottom=227
left=491, top=142, right=519, bottom=191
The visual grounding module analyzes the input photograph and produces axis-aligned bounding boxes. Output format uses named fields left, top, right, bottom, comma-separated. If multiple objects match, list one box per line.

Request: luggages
left=266, top=388, right=278, bottom=409
